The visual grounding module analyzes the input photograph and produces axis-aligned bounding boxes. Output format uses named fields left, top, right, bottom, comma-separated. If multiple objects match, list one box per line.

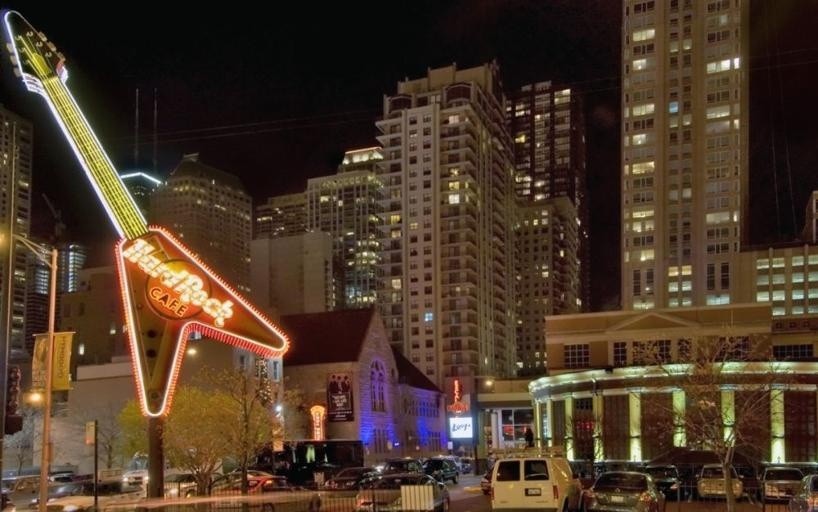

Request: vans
left=119, top=448, right=223, bottom=495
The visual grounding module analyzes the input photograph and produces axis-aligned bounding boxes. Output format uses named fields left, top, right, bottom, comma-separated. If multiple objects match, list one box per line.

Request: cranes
left=40, top=193, right=66, bottom=226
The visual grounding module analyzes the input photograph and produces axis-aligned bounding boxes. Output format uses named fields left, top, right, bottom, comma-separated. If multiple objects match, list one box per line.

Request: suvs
left=491, top=454, right=585, bottom=512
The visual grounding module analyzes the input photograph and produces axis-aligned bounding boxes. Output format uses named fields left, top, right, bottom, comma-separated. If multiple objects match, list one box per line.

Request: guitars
left=4, top=11, right=289, bottom=417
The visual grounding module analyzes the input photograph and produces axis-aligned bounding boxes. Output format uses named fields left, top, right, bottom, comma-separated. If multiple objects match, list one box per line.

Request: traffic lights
left=6, top=363, right=23, bottom=436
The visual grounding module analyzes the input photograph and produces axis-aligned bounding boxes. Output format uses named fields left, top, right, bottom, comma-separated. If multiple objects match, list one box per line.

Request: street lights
left=0, top=231, right=58, bottom=512
left=472, top=375, right=497, bottom=475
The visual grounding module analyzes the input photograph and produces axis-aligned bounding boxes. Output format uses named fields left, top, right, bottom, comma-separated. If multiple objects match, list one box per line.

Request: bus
left=250, top=439, right=364, bottom=469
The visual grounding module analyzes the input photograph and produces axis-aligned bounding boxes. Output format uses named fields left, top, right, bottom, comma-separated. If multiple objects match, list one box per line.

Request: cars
left=165, top=470, right=322, bottom=512
left=325, top=456, right=473, bottom=512
left=480, top=464, right=493, bottom=496
left=644, top=464, right=683, bottom=498
left=1, top=465, right=94, bottom=508
left=582, top=471, right=666, bottom=512
left=759, top=467, right=807, bottom=503
left=696, top=464, right=744, bottom=500
left=788, top=474, right=818, bottom=512
